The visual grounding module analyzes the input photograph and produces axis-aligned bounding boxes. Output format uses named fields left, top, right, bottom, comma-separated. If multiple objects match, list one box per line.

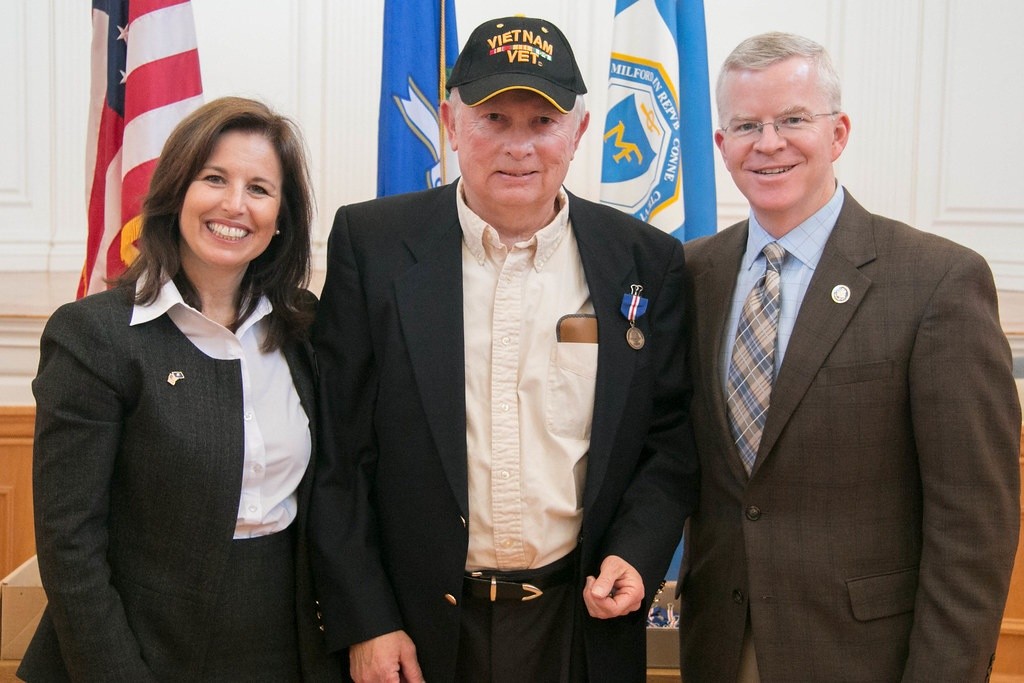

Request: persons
left=13, top=92, right=331, bottom=683
left=663, top=27, right=1024, bottom=683
left=301, top=13, right=697, bottom=683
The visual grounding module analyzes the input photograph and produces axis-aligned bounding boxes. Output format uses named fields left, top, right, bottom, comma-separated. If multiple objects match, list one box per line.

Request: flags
left=596, top=1, right=724, bottom=644
left=370, top=0, right=472, bottom=207
left=75, top=1, right=215, bottom=309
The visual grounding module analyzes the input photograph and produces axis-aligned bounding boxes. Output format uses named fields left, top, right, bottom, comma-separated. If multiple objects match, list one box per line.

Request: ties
left=724, top=241, right=787, bottom=483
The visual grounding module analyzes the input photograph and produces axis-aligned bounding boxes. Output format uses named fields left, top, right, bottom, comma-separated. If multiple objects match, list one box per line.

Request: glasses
left=721, top=111, right=837, bottom=135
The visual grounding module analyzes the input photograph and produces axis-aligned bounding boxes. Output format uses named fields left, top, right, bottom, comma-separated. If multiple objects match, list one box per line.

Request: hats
left=446, top=9, right=587, bottom=114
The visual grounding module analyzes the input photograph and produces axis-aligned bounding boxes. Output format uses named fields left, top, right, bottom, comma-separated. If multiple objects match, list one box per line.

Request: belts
left=460, top=538, right=584, bottom=603
